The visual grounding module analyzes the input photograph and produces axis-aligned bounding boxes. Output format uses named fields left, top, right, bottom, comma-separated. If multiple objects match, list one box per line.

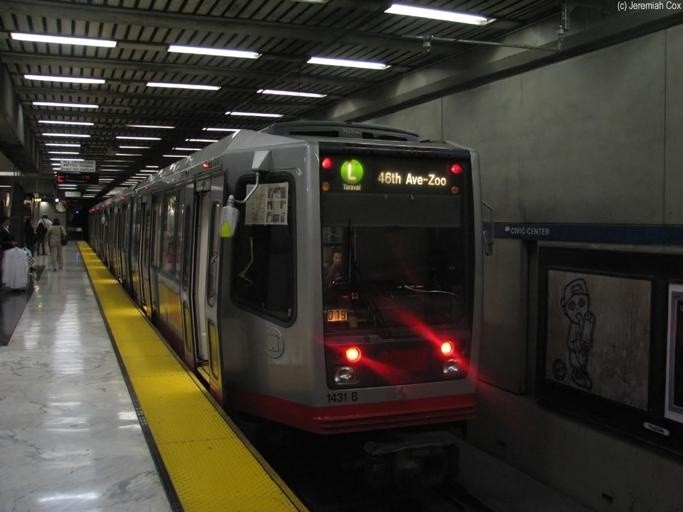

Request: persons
left=0, top=213, right=19, bottom=292
left=34, top=214, right=52, bottom=256
left=43, top=218, right=66, bottom=272
left=22, top=216, right=34, bottom=254
left=321, top=245, right=341, bottom=284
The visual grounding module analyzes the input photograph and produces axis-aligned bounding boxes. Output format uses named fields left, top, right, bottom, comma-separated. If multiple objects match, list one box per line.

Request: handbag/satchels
left=60, top=227, right=68, bottom=246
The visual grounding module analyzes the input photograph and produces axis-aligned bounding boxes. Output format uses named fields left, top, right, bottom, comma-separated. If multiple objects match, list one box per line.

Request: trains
left=89, top=119, right=494, bottom=434
